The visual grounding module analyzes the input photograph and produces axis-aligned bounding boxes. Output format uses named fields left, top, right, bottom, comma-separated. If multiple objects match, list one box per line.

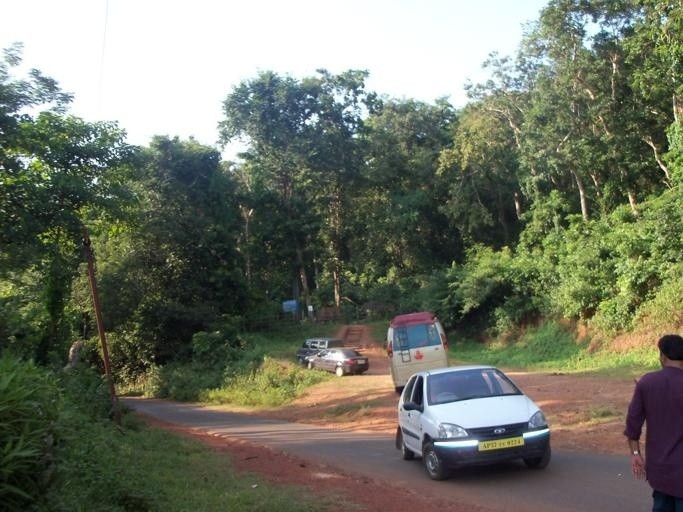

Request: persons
left=623, top=334, right=682, bottom=512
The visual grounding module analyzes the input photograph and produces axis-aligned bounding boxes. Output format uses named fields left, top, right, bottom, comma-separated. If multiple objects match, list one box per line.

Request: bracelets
left=631, top=449, right=641, bottom=456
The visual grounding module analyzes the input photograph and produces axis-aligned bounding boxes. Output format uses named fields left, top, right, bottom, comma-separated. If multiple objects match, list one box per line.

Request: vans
left=383, top=312, right=449, bottom=392
left=295, top=338, right=345, bottom=367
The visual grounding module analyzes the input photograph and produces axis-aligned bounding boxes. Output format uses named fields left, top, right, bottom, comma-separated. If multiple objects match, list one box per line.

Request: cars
left=303, top=347, right=369, bottom=377
left=396, top=364, right=552, bottom=480
